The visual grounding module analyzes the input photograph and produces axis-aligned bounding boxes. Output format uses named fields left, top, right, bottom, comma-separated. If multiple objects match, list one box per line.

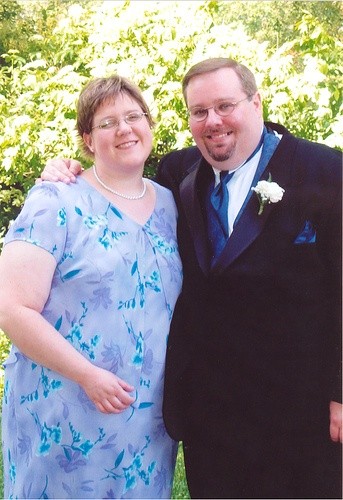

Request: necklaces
left=94, top=166, right=146, bottom=200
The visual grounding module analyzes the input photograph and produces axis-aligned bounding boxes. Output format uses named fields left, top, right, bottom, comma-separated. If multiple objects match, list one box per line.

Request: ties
left=206, top=130, right=264, bottom=272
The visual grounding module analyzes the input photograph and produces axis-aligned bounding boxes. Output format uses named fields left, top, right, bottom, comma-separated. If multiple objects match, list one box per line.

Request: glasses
left=186, top=96, right=248, bottom=122
left=92, top=111, right=148, bottom=130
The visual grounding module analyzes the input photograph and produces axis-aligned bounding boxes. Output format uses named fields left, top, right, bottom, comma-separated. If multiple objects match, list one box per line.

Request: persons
left=0, top=75, right=182, bottom=500
left=35, top=58, right=343, bottom=500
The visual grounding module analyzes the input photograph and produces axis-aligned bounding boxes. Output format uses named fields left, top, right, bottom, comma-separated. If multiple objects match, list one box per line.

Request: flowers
left=250, top=172, right=286, bottom=215
